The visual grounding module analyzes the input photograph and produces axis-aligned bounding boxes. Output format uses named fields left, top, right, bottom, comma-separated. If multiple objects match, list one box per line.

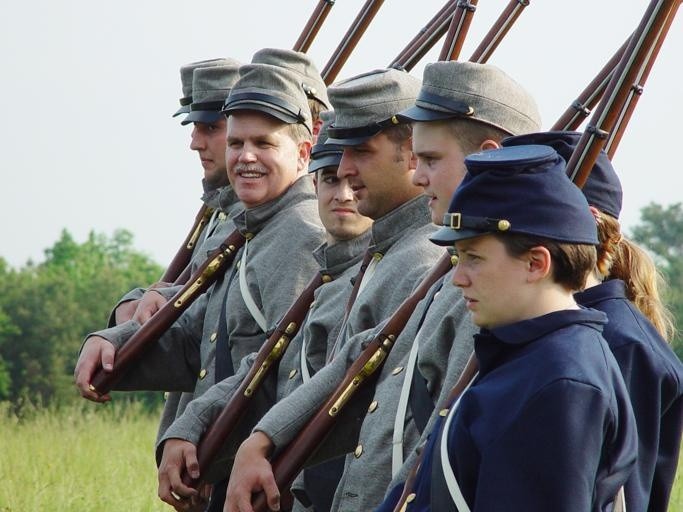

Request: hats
left=221, top=64, right=313, bottom=126
left=251, top=46, right=330, bottom=109
left=326, top=64, right=422, bottom=148
left=181, top=64, right=241, bottom=125
left=306, top=111, right=346, bottom=173
left=172, top=58, right=239, bottom=119
left=429, top=145, right=601, bottom=247
left=501, top=127, right=624, bottom=219
left=394, top=60, right=544, bottom=136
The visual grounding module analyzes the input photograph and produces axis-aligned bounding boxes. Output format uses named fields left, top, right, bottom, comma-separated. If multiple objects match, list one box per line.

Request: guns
left=170, top=1, right=536, bottom=512
left=156, top=1, right=334, bottom=292
left=320, top=1, right=383, bottom=88
left=392, top=0, right=682, bottom=512
left=249, top=27, right=632, bottom=512
left=598, top=0, right=682, bottom=164
left=90, top=1, right=461, bottom=398
left=344, top=1, right=481, bottom=318
